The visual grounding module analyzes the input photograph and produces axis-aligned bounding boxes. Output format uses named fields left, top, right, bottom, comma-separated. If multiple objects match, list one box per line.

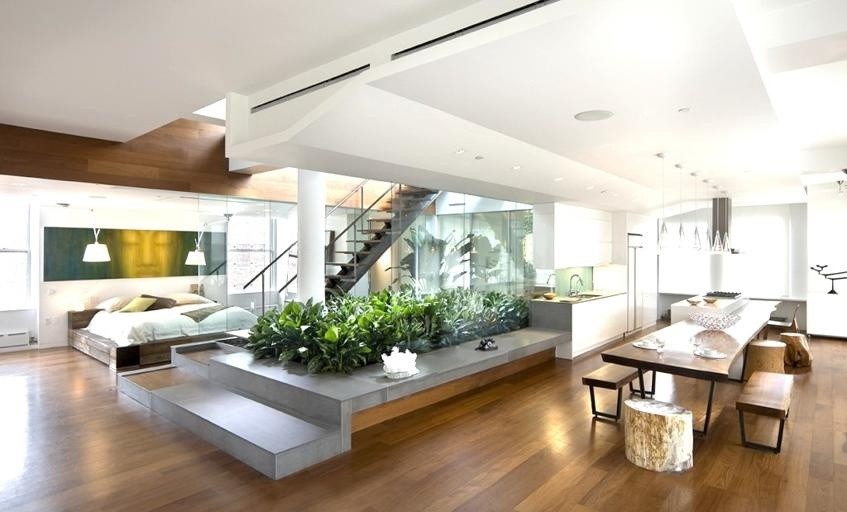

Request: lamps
left=184, top=213, right=233, bottom=267
left=81, top=224, right=112, bottom=264
left=645, top=150, right=733, bottom=256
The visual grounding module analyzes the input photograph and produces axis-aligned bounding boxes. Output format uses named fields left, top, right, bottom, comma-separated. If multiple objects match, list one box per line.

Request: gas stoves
left=702, top=290, right=741, bottom=299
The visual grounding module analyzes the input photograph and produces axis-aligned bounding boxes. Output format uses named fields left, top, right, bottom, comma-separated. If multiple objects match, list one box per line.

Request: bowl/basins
left=703, top=298, right=716, bottom=304
left=543, top=293, right=556, bottom=300
left=686, top=299, right=701, bottom=306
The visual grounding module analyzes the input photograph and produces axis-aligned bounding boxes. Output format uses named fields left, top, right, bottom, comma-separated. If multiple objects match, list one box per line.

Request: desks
left=599, top=302, right=781, bottom=438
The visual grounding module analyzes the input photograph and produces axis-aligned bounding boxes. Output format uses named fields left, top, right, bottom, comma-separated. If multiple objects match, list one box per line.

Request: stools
left=779, top=331, right=814, bottom=369
left=741, top=338, right=786, bottom=382
left=735, top=370, right=796, bottom=456
left=622, top=395, right=695, bottom=476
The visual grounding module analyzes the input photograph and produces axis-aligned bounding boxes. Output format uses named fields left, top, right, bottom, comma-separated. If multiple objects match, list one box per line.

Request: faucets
left=569, top=273, right=584, bottom=289
left=546, top=273, right=556, bottom=283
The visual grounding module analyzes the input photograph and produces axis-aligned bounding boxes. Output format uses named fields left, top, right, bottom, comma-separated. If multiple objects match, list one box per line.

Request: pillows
left=91, top=291, right=212, bottom=314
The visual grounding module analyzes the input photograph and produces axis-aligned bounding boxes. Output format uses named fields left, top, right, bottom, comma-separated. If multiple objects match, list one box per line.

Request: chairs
left=762, top=303, right=802, bottom=341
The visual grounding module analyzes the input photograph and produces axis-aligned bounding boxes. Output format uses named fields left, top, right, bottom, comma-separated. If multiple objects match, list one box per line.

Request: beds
left=64, top=290, right=258, bottom=374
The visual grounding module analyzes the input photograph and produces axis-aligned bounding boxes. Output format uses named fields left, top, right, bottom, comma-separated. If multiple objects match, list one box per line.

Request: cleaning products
left=200, top=285, right=204, bottom=296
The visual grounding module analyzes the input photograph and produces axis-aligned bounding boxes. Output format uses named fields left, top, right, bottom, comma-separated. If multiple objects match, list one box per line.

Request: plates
left=631, top=340, right=658, bottom=349
left=693, top=349, right=728, bottom=359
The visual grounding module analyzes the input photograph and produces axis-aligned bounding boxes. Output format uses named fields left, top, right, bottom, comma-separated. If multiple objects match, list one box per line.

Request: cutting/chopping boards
left=559, top=297, right=579, bottom=302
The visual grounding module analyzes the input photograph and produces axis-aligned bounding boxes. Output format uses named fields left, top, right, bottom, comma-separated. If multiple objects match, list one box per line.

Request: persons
left=101, top=228, right=183, bottom=277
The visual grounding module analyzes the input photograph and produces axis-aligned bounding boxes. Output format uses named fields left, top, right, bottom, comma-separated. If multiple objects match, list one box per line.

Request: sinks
left=573, top=294, right=601, bottom=298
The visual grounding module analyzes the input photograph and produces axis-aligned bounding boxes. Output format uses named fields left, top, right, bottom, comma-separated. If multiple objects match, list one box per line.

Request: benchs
left=580, top=362, right=658, bottom=424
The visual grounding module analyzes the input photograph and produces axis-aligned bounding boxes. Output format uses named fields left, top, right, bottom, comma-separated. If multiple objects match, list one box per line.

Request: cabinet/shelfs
left=529, top=295, right=626, bottom=361
left=532, top=201, right=612, bottom=269
